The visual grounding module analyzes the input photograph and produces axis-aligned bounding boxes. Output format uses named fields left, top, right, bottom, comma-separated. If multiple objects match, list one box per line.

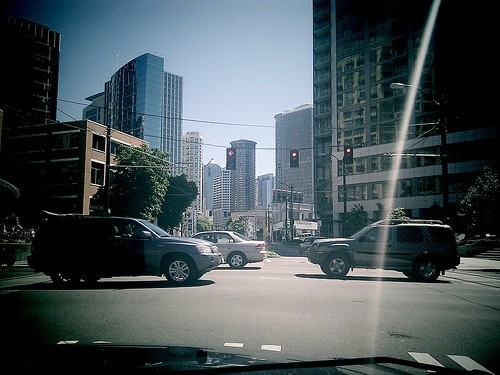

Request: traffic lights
left=345, top=147, right=354, bottom=164
left=226, top=148, right=236, bottom=170
left=290, top=149, right=299, bottom=168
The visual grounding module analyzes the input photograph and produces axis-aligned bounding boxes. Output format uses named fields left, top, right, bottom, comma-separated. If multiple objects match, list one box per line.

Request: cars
left=191, top=230, right=268, bottom=269
left=294, top=233, right=325, bottom=254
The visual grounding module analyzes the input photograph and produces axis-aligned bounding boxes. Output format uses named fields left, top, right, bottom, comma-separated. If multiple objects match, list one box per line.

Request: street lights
left=280, top=182, right=294, bottom=241
left=390, top=83, right=451, bottom=220
left=317, top=152, right=347, bottom=214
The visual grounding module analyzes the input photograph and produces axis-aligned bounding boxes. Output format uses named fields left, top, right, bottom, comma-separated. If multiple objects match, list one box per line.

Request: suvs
left=308, top=219, right=460, bottom=283
left=28, top=210, right=224, bottom=287
